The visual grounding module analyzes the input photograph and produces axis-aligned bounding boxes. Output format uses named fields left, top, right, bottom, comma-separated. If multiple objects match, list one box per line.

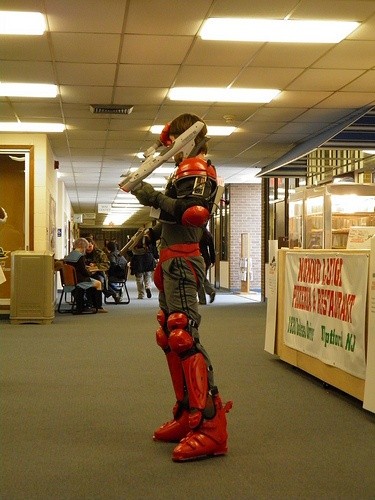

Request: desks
left=55, top=267, right=108, bottom=308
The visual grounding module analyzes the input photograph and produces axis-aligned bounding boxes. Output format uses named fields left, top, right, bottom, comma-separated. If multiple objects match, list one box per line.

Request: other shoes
left=172, top=387, right=228, bottom=462
left=96, top=308, right=111, bottom=314
left=153, top=388, right=194, bottom=443
left=210, top=291, right=216, bottom=304
left=146, top=288, right=152, bottom=298
left=197, top=301, right=207, bottom=305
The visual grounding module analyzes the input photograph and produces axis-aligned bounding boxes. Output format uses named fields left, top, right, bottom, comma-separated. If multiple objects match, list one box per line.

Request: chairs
left=104, top=262, right=130, bottom=305
left=58, top=263, right=98, bottom=315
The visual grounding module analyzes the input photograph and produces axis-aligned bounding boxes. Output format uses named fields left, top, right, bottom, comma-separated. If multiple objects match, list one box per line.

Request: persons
left=130, top=114, right=234, bottom=461
left=63, top=225, right=217, bottom=313
